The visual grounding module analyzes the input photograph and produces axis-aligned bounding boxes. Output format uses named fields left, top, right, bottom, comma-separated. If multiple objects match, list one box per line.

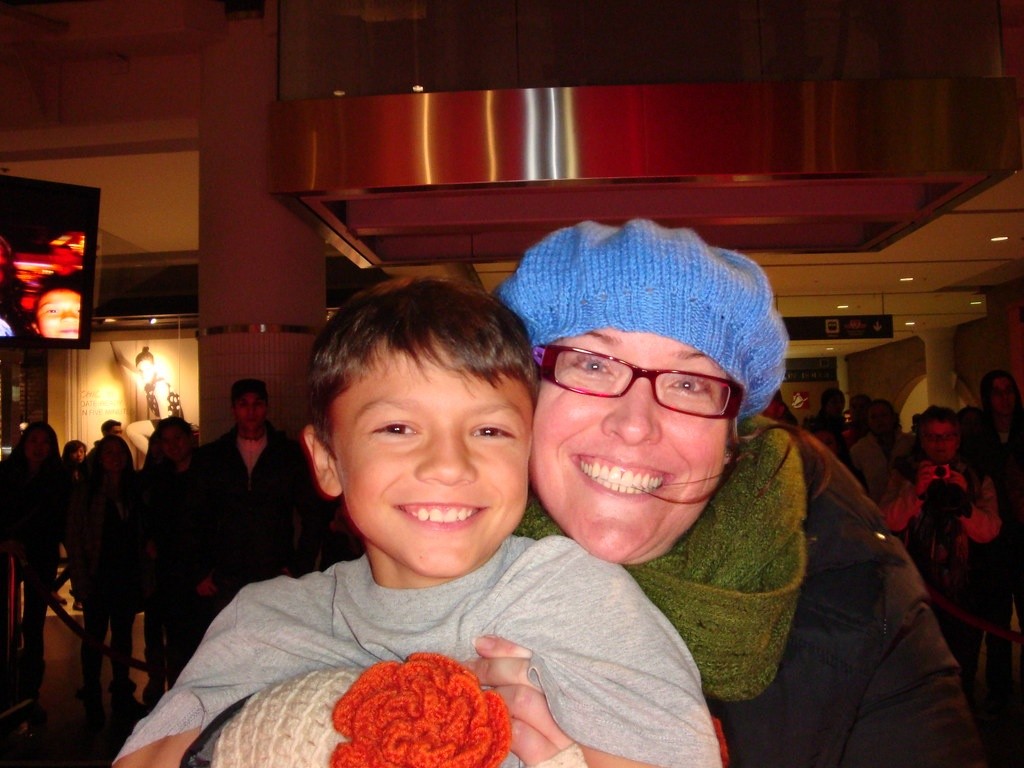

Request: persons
left=0, top=378, right=362, bottom=723
left=761, top=369, right=1024, bottom=721
left=109, top=338, right=185, bottom=458
left=114, top=218, right=986, bottom=767
left=112, top=275, right=723, bottom=768
left=0, top=233, right=84, bottom=339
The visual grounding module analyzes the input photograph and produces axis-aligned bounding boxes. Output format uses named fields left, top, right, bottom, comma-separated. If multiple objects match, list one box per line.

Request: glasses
left=530, top=344, right=746, bottom=421
left=919, top=430, right=960, bottom=443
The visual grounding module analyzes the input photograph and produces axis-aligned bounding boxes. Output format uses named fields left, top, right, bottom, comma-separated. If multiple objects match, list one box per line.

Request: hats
left=231, top=377, right=270, bottom=406
left=177, top=653, right=512, bottom=768
left=491, top=217, right=789, bottom=426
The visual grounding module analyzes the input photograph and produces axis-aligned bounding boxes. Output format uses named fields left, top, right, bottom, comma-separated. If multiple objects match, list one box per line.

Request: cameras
left=926, top=464, right=950, bottom=479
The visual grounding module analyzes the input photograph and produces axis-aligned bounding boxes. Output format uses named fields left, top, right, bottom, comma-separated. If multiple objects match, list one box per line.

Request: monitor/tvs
left=0, top=176, right=102, bottom=350
